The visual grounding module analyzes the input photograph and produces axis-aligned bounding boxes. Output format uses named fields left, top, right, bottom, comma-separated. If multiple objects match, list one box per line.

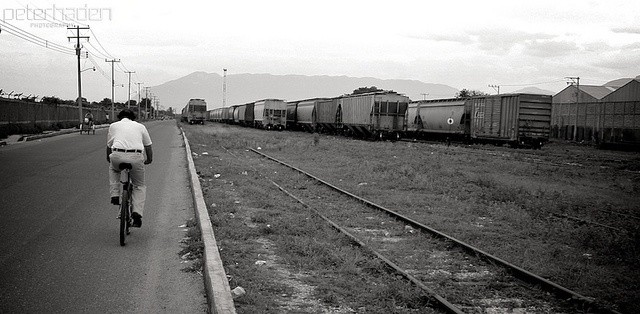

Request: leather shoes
left=111, top=196, right=119, bottom=205
left=131, top=212, right=142, bottom=227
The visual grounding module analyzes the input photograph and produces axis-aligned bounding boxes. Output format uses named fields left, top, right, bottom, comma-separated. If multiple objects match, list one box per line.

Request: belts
left=113, top=148, right=142, bottom=152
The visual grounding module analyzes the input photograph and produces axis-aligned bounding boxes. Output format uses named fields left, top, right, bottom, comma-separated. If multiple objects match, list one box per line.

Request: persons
left=85, top=110, right=94, bottom=119
left=106, top=109, right=153, bottom=227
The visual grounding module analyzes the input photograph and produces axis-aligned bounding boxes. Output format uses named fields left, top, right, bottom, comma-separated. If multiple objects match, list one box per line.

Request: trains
left=554, top=101, right=636, bottom=141
left=181, top=98, right=209, bottom=126
left=404, top=92, right=553, bottom=151
left=209, top=98, right=287, bottom=131
left=286, top=85, right=409, bottom=143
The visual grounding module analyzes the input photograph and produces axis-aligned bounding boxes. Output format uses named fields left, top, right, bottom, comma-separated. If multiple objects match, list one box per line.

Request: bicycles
left=106, top=156, right=151, bottom=246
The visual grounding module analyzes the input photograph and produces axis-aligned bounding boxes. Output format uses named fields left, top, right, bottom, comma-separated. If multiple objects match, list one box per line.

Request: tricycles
left=79, top=115, right=95, bottom=136
